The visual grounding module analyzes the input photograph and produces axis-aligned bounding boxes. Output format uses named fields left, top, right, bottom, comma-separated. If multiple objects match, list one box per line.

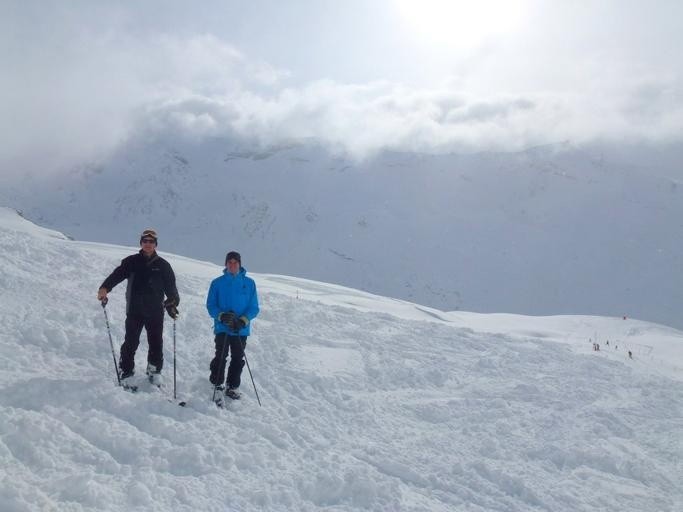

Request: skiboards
left=157, top=384, right=187, bottom=406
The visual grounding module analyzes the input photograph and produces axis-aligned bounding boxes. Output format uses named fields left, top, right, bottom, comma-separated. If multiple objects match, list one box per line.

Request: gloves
left=220, top=310, right=236, bottom=326
left=230, top=318, right=245, bottom=332
left=162, top=295, right=180, bottom=319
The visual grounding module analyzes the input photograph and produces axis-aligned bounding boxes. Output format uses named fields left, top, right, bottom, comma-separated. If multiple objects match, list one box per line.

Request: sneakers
left=210, top=385, right=226, bottom=411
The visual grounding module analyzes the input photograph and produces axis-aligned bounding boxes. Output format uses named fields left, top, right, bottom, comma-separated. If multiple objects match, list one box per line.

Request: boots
left=225, top=383, right=242, bottom=400
left=116, top=369, right=135, bottom=389
left=146, top=363, right=164, bottom=388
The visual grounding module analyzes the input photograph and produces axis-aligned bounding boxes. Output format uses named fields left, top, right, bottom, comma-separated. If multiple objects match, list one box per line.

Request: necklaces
left=206, top=250, right=259, bottom=399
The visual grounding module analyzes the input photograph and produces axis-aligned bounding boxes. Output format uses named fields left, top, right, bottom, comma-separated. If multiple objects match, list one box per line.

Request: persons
left=97, top=227, right=180, bottom=388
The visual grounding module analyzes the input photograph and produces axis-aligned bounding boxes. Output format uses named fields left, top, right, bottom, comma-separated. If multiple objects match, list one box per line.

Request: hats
left=225, top=251, right=241, bottom=266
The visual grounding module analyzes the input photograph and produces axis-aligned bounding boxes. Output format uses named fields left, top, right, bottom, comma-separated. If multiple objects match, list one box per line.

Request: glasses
left=140, top=228, right=158, bottom=244
